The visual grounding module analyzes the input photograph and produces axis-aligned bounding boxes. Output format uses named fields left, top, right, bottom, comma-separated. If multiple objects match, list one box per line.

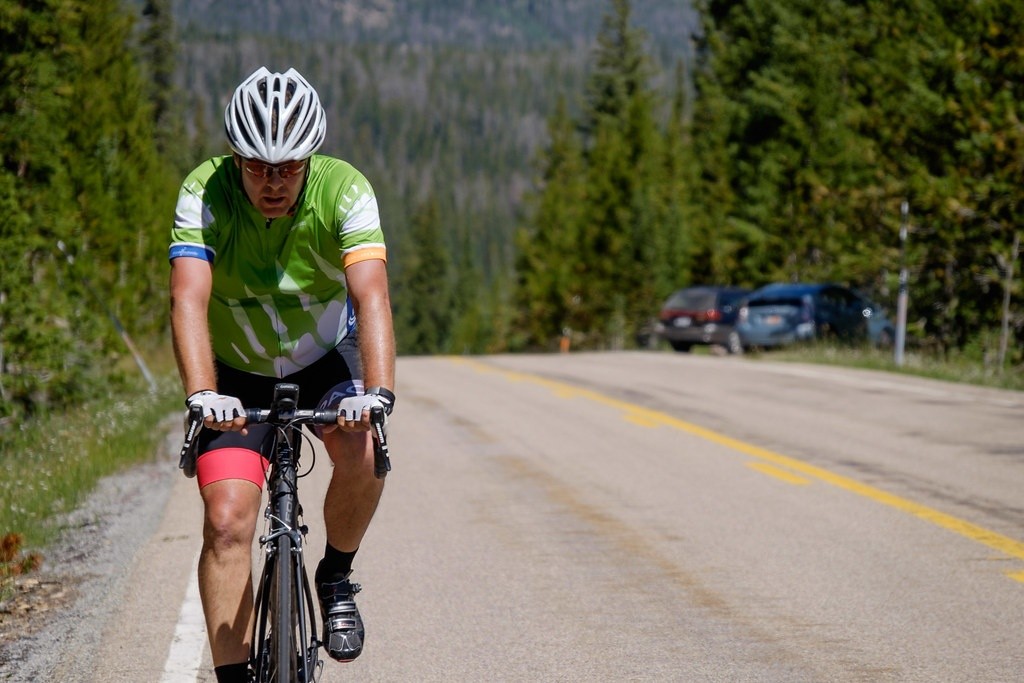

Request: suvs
left=653, top=287, right=748, bottom=353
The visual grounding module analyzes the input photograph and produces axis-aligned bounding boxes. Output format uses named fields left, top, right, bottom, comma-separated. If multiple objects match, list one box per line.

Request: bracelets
left=364, top=385, right=395, bottom=404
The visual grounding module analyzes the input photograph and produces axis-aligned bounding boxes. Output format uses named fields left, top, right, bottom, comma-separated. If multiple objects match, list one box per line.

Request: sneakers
left=314, top=558, right=365, bottom=663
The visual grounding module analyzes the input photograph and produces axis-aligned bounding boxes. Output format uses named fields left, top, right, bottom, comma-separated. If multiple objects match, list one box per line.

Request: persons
left=170, top=67, right=396, bottom=683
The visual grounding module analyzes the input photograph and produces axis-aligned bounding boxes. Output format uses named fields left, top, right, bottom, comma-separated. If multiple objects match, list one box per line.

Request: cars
left=736, top=280, right=895, bottom=354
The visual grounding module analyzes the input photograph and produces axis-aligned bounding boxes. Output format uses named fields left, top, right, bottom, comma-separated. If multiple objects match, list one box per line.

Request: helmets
left=223, top=66, right=327, bottom=168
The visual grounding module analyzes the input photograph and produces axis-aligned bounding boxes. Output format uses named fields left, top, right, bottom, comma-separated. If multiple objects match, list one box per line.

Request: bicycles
left=179, top=383, right=392, bottom=683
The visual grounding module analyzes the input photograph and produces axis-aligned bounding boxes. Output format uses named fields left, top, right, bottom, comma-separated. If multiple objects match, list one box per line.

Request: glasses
left=238, top=158, right=308, bottom=178
